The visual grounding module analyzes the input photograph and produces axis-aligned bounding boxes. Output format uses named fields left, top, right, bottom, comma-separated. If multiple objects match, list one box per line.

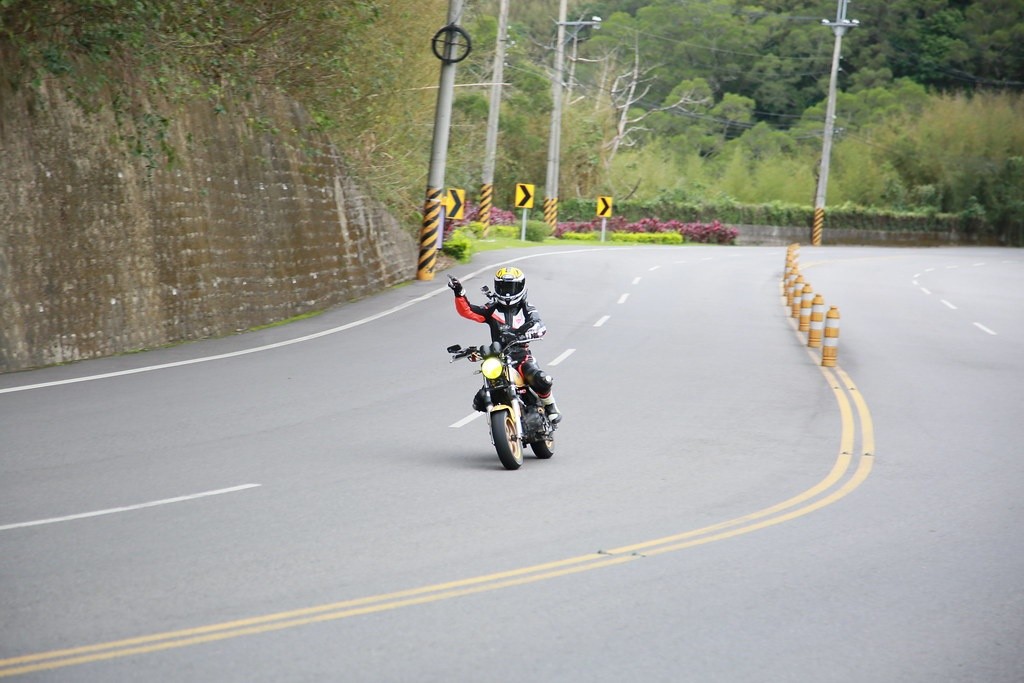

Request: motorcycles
left=446, top=332, right=557, bottom=470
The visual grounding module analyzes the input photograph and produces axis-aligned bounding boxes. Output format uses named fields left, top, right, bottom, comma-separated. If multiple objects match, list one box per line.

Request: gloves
left=446, top=273, right=468, bottom=297
left=524, top=328, right=542, bottom=339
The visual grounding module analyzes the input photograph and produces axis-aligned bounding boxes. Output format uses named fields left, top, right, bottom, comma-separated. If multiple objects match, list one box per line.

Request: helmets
left=494, top=266, right=528, bottom=306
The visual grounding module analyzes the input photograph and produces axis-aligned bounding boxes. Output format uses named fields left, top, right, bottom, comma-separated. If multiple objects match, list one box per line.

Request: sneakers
left=543, top=401, right=563, bottom=424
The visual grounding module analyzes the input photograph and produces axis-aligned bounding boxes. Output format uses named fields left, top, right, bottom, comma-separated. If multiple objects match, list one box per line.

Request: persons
left=445, top=265, right=562, bottom=425
